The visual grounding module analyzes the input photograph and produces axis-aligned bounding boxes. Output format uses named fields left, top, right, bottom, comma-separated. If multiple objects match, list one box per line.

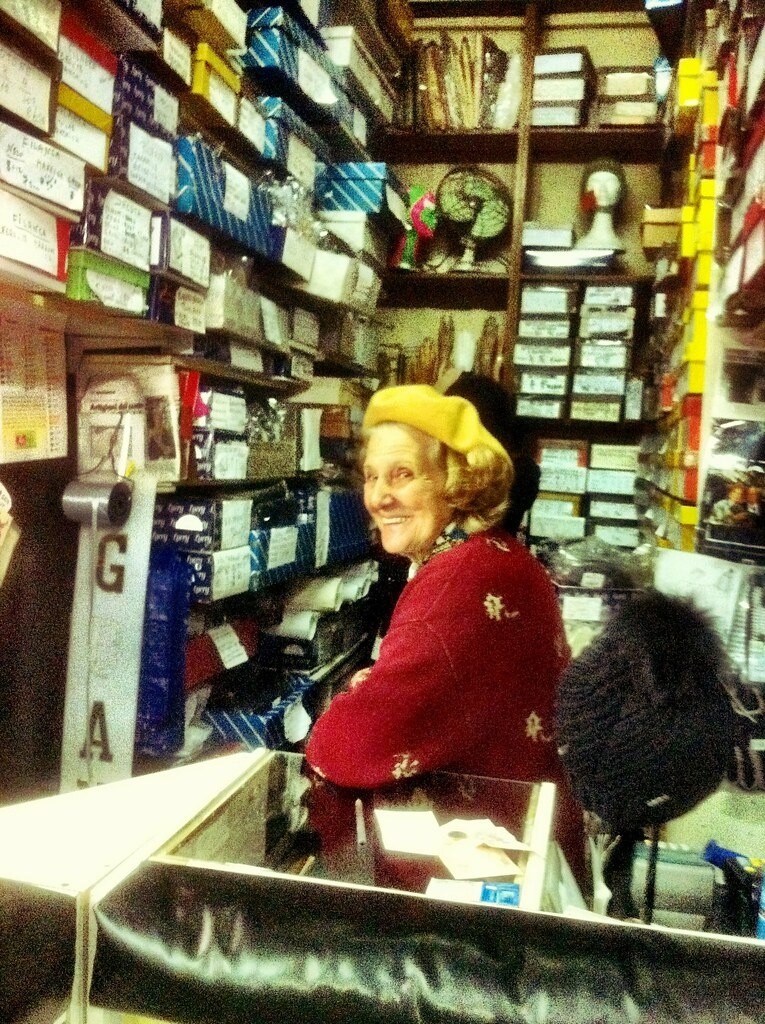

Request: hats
left=361, top=384, right=514, bottom=470
left=552, top=594, right=739, bottom=827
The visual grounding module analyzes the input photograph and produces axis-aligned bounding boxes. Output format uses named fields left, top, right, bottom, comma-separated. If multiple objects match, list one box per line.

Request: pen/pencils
left=355, top=797, right=366, bottom=845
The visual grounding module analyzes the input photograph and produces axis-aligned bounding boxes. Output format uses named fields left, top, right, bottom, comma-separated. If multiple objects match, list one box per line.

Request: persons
left=305, top=382, right=589, bottom=893
left=383, top=372, right=541, bottom=630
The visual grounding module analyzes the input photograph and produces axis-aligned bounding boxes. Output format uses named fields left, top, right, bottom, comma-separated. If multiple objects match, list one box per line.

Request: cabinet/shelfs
left=1, top=0, right=764, bottom=1023
